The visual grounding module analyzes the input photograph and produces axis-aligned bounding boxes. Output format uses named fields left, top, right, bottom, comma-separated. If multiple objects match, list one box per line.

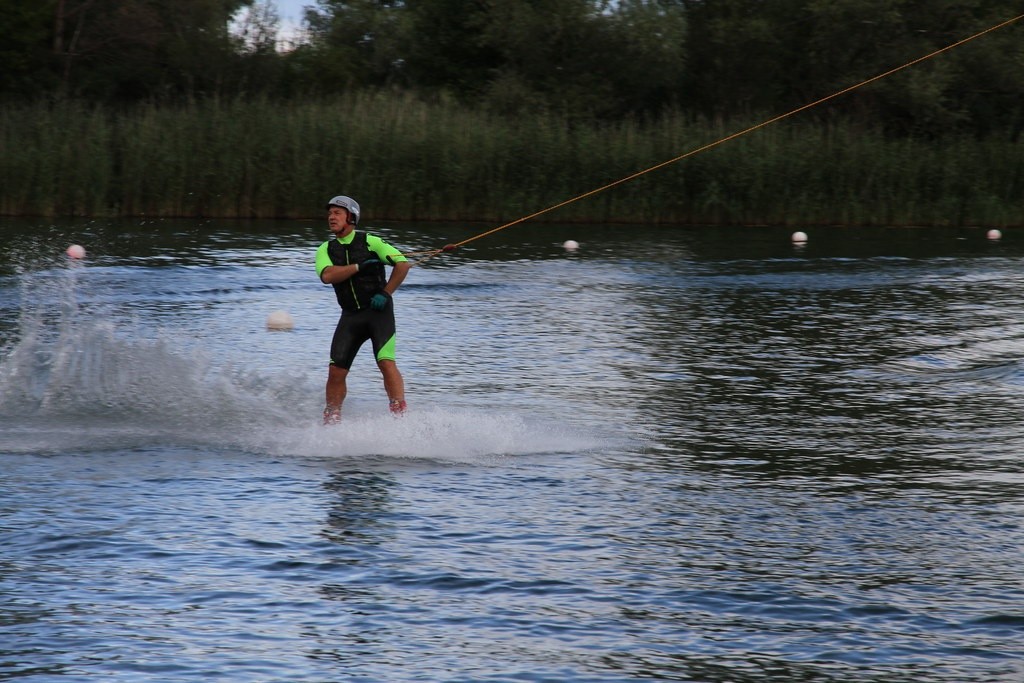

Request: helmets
left=327, top=196, right=360, bottom=225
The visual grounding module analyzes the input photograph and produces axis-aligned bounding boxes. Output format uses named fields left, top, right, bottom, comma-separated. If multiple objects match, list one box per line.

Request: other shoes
left=322, top=409, right=340, bottom=425
left=389, top=401, right=407, bottom=418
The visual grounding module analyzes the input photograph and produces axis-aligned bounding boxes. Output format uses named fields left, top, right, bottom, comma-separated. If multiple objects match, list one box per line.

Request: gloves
left=358, top=259, right=390, bottom=277
left=370, top=291, right=392, bottom=312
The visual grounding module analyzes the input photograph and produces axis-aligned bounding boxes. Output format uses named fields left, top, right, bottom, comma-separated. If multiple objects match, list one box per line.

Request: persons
left=315, top=196, right=410, bottom=425
